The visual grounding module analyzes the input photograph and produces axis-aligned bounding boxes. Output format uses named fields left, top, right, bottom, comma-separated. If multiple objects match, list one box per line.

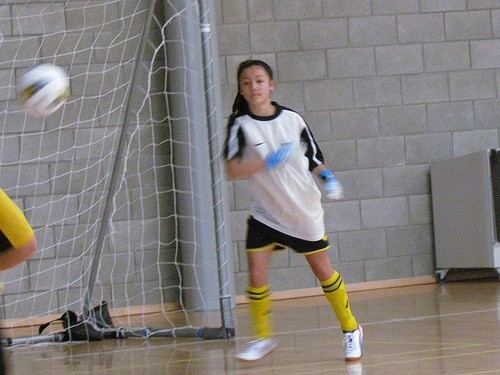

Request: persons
left=222, top=59, right=364, bottom=363
left=1, top=188, right=37, bottom=271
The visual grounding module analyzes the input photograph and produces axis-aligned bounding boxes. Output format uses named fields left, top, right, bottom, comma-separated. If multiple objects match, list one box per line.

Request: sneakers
left=341, top=324, right=363, bottom=361
left=235, top=337, right=280, bottom=362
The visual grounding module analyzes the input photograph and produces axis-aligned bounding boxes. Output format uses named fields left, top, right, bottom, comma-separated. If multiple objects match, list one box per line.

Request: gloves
left=265, top=143, right=292, bottom=168
left=319, top=170, right=344, bottom=201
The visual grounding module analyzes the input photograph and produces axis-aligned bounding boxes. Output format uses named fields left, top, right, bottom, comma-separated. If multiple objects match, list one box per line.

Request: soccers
left=18, top=63, right=70, bottom=116
